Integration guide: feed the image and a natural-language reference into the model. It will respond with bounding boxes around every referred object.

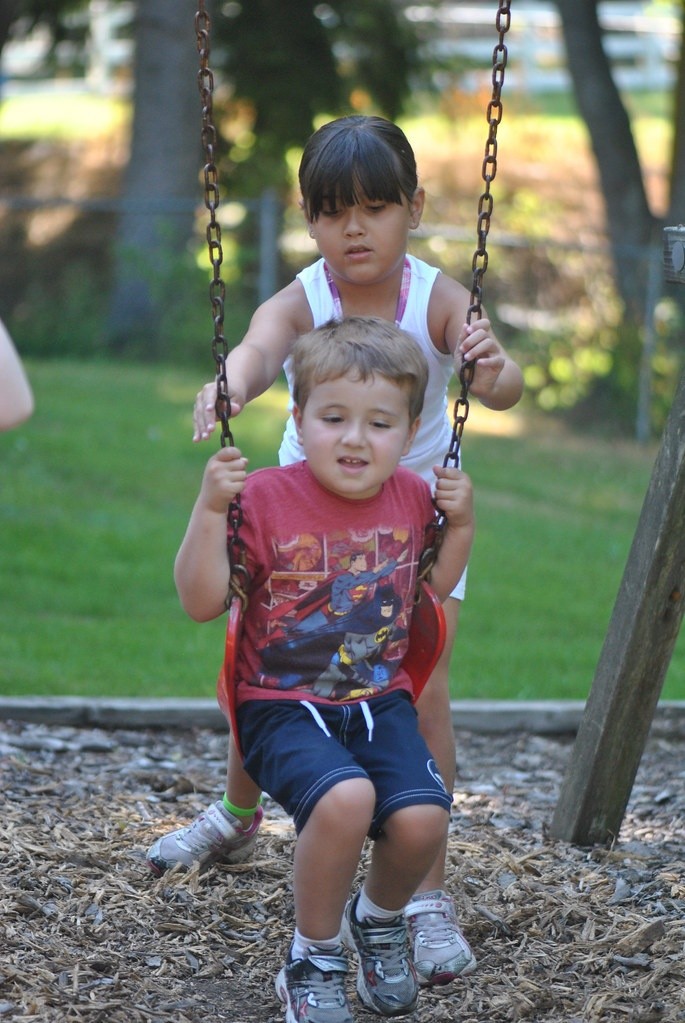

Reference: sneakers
[274,939,351,1023]
[340,891,419,1017]
[144,801,262,875]
[404,889,476,986]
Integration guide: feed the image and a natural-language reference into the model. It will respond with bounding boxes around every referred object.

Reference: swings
[191,0,513,760]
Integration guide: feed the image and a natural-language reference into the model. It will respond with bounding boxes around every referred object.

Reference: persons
[0,318,36,434]
[145,113,523,990]
[172,316,474,1023]
